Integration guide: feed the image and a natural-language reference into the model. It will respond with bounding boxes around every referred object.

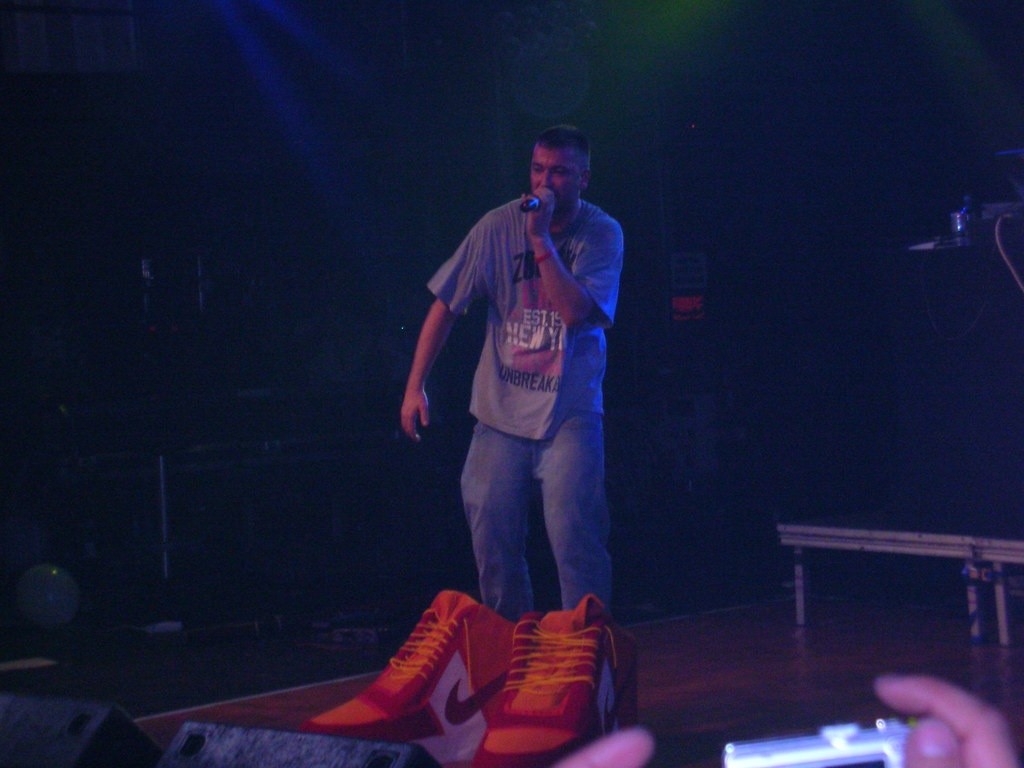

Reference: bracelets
[534,248,554,263]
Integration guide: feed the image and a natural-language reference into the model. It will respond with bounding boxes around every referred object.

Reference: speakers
[0,693,442,768]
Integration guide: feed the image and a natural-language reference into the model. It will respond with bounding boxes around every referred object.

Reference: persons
[552,675,1020,768]
[400,126,624,617]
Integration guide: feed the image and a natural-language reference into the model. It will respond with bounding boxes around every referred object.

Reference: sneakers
[297,589,516,742]
[472,593,638,768]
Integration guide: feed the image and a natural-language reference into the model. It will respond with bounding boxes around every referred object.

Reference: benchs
[775,514,1024,647]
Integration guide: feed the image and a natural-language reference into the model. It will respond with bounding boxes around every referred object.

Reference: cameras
[721,722,909,768]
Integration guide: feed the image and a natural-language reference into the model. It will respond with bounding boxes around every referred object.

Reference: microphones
[520,187,555,212]
